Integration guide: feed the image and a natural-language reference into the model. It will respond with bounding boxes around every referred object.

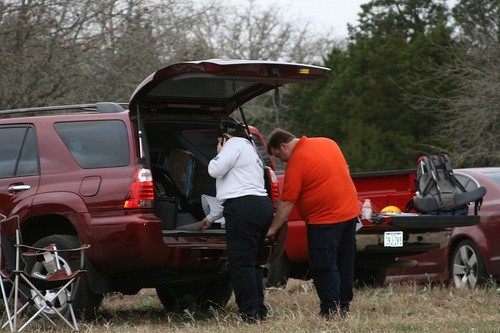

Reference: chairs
[0,215,91,333]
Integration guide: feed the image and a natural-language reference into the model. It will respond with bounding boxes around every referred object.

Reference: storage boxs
[156,196,178,230]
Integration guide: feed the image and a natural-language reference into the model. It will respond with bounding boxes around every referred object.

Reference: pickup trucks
[242,124,487,290]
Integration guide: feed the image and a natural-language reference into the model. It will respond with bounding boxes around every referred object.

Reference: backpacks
[405,152,487,215]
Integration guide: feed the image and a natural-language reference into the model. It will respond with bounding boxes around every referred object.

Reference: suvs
[0,58,331,323]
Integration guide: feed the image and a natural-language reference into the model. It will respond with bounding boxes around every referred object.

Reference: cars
[383,167,500,291]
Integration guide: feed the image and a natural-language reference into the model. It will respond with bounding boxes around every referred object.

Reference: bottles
[362,199,372,220]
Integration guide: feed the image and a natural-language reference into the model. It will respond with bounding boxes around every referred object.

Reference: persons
[200,117,275,325]
[264,129,363,321]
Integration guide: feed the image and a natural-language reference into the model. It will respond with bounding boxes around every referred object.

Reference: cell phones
[221,135,229,146]
